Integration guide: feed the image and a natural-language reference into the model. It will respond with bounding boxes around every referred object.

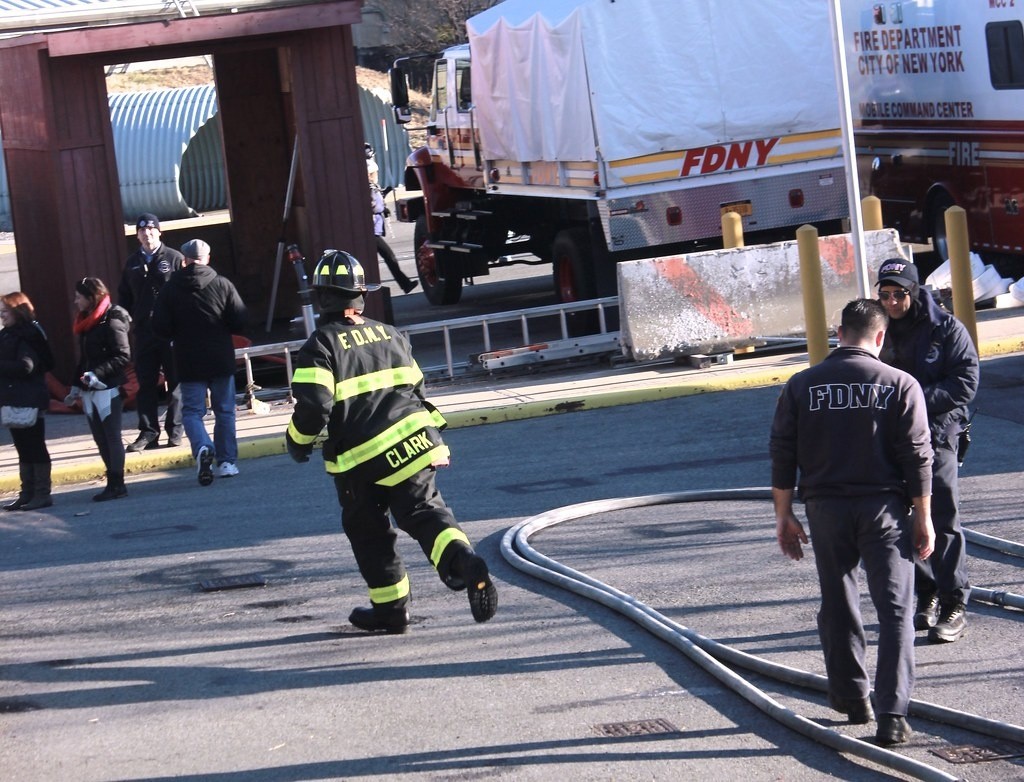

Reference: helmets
[310,249,381,291]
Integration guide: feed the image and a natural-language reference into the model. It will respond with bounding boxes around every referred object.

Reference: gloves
[287,440,312,463]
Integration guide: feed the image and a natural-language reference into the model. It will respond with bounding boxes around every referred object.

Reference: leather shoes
[876,714,912,742]
[463,555,499,623]
[349,607,410,634]
[848,697,876,723]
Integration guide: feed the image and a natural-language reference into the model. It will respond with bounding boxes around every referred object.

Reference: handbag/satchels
[1,406,38,426]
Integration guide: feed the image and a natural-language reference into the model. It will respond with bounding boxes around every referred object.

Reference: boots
[3,463,53,510]
[94,470,128,501]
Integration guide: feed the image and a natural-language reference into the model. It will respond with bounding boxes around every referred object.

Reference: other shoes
[404,281,418,293]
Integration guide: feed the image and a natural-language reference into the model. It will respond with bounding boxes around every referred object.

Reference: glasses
[878,288,909,300]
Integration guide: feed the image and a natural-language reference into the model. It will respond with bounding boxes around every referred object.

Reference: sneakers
[913,593,941,629]
[127,437,159,452]
[168,434,182,446]
[928,603,967,642]
[197,446,214,487]
[218,462,239,475]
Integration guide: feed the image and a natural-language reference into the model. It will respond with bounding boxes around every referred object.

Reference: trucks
[392,0,861,324]
[836,0,1024,251]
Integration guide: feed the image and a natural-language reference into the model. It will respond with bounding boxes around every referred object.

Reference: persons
[770,299,935,742]
[154,238,248,486]
[287,250,499,632]
[366,157,418,293]
[876,258,979,640]
[119,213,187,450]
[0,278,132,511]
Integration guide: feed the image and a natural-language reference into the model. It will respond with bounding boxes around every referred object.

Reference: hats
[181,239,210,259]
[136,213,160,232]
[366,159,379,173]
[874,258,919,290]
